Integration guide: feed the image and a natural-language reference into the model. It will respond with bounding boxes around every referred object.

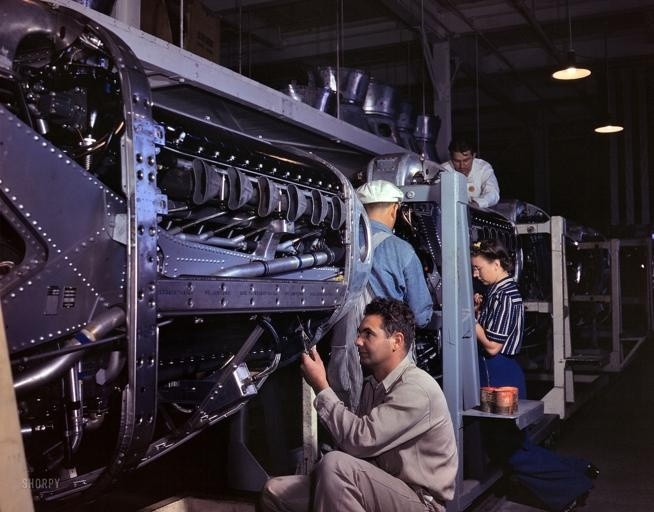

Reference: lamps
[548,0,594,82]
[589,31,624,135]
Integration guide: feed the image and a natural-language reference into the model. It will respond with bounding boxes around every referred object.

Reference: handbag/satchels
[506,445,595,511]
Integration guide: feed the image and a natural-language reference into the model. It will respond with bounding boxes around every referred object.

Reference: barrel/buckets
[480,387,497,412]
[498,387,519,412]
[496,390,513,416]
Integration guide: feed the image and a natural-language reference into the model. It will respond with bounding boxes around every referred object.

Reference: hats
[355,180,404,205]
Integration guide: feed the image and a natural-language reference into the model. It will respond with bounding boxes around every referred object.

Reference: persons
[261,296,459,511]
[470,237,527,400]
[432,138,502,241]
[326,179,434,415]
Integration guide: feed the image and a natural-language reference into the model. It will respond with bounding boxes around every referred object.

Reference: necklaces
[488,270,507,296]
